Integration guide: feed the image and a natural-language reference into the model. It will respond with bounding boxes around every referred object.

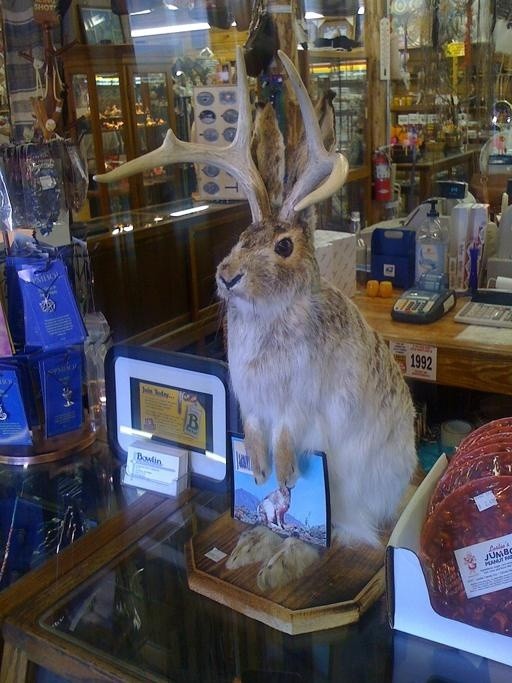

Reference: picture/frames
[76,2,127,45]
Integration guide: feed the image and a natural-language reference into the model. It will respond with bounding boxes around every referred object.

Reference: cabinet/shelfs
[60,43,187,220]
[388,39,511,214]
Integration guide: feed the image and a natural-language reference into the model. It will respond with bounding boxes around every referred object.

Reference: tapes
[441,419,472,448]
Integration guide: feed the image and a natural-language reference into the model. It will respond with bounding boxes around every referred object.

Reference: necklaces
[49,367,75,408]
[0,381,13,420]
[30,274,66,313]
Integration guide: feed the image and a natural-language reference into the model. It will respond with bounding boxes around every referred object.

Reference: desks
[349,283,511,396]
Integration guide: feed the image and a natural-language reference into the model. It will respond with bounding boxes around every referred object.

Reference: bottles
[348,211,368,291]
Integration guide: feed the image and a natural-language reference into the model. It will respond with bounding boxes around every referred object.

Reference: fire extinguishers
[372,144,395,201]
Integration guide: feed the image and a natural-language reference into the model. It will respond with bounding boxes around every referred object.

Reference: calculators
[455,288,512,326]
